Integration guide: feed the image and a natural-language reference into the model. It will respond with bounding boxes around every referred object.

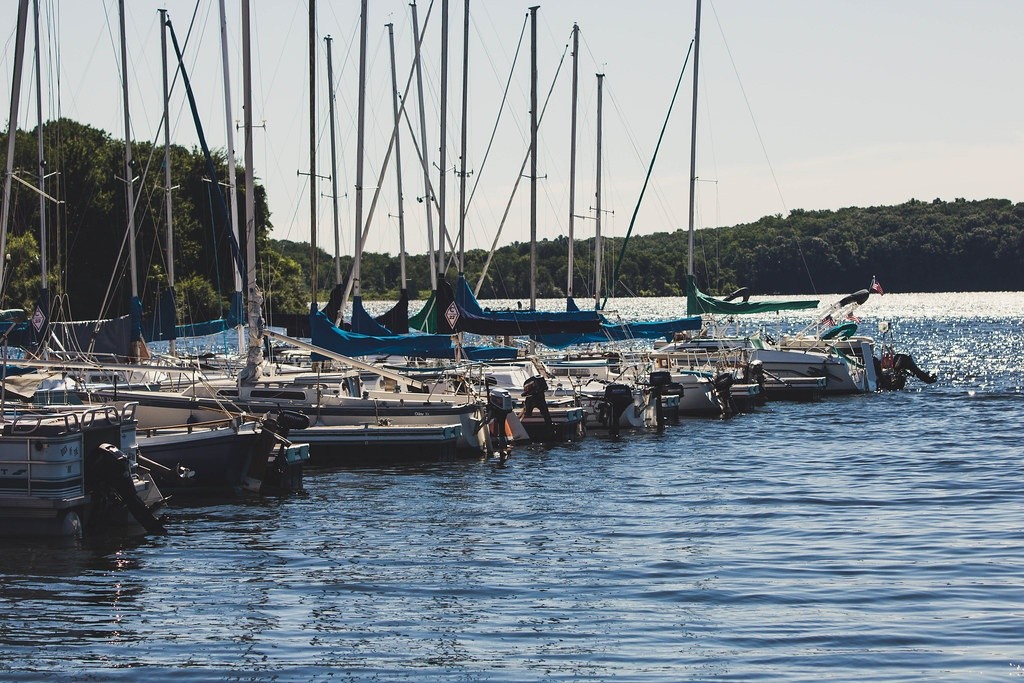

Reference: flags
[871,279,884,295]
[822,314,835,328]
[845,312,862,323]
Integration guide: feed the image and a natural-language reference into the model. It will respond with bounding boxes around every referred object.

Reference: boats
[0,0,931,534]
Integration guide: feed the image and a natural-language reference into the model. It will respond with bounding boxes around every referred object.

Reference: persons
[522,373,556,435]
